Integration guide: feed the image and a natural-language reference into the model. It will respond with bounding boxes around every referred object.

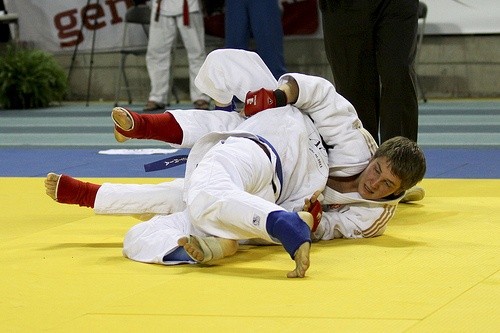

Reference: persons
[224,0,287,103]
[142,0,211,110]
[319,0,426,203]
[123,48,329,278]
[45,73,426,246]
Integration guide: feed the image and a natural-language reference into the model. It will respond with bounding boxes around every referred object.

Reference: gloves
[303,199,322,233]
[245,87,276,118]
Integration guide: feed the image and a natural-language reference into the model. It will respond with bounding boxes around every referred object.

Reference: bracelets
[274,89,287,107]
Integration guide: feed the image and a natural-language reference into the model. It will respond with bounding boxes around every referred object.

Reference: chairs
[410,1,428,105]
[114,5,180,107]
